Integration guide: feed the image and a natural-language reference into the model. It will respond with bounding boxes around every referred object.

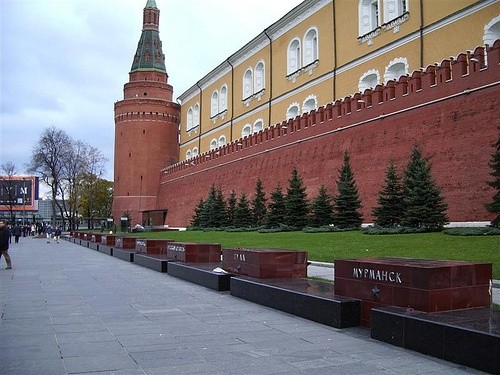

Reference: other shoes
[5,267,12,270]
[57,242,59,244]
[47,241,50,244]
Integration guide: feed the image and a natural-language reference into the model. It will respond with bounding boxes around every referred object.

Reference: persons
[5,221,61,243]
[0,220,12,269]
